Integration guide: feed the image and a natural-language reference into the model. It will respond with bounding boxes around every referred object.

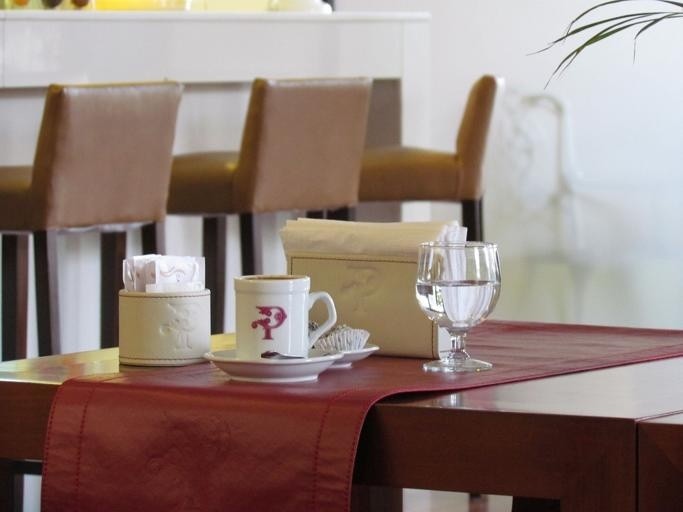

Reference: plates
[313,342,380,369]
[204,348,342,383]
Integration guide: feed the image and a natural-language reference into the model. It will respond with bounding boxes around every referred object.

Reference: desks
[0,12,439,350]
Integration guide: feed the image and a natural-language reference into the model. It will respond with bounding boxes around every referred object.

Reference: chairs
[0,81,183,359]
[142,74,373,335]
[325,74,498,242]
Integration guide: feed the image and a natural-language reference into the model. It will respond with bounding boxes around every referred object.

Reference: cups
[234,274,337,360]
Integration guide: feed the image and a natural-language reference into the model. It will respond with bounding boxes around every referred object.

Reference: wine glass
[414,241,502,374]
